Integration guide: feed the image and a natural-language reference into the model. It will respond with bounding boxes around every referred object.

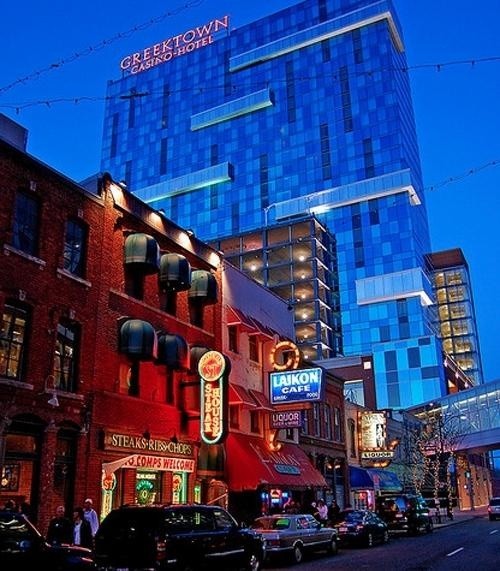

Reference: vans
[374,493,433,534]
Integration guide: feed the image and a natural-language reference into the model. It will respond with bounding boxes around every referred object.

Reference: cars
[326,510,391,547]
[239,514,338,561]
[0,511,94,570]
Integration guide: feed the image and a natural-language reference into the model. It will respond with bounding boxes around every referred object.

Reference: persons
[82,498,99,539]
[46,506,73,545]
[71,508,92,550]
[3,500,17,513]
[18,503,31,519]
[271,496,340,527]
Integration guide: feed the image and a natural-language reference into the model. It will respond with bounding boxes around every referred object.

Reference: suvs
[487,497,500,521]
[94,506,267,571]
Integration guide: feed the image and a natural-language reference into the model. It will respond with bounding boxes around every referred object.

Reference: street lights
[438,416,469,517]
[326,462,342,504]
